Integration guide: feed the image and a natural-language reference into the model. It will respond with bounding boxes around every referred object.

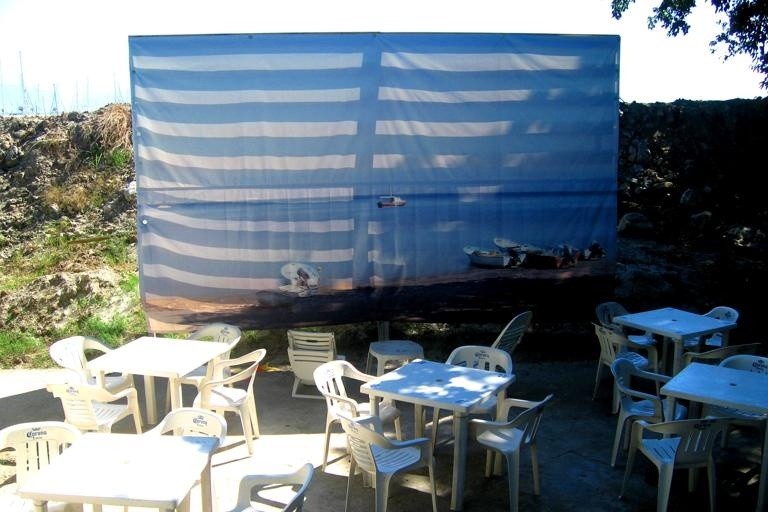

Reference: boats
[280,260,322,293]
[463,236,607,269]
[256,286,305,309]
[378,184,405,208]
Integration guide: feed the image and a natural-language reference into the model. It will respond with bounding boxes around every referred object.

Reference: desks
[359,358,516,511]
[85,336,229,434]
[19,433,219,512]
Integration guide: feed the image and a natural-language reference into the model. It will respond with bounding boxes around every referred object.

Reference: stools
[366,339,424,403]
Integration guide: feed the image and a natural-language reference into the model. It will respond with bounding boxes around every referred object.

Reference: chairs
[121,408,227,512]
[165,323,241,414]
[287,331,346,401]
[593,294,767,512]
[458,310,533,367]
[0,421,83,512]
[50,336,144,428]
[313,361,402,489]
[193,348,267,455]
[229,463,314,512]
[467,394,554,511]
[337,414,437,512]
[432,345,512,438]
[36,368,141,434]
